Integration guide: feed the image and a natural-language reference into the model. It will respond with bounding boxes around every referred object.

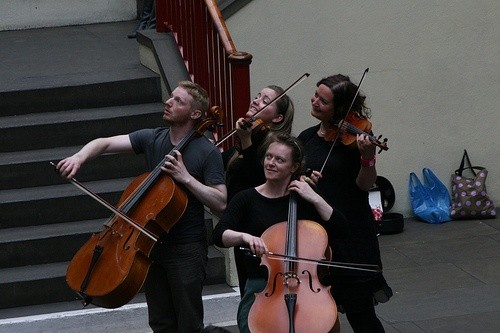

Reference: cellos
[246,170,341,333]
[65,105,224,310]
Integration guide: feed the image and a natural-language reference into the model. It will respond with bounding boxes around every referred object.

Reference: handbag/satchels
[409,167,451,223]
[450,149,496,219]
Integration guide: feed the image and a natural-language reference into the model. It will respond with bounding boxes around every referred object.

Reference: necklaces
[320,123,326,135]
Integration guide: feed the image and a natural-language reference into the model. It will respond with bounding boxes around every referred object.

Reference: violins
[237,116,269,144]
[323,108,390,154]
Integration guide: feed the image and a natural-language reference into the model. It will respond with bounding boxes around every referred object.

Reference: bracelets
[361,154,376,167]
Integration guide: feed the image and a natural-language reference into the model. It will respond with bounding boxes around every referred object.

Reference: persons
[296,75,393,333]
[56,81,228,333]
[208,85,294,300]
[210,130,342,333]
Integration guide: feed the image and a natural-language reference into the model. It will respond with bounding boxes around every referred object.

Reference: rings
[305,177,310,181]
